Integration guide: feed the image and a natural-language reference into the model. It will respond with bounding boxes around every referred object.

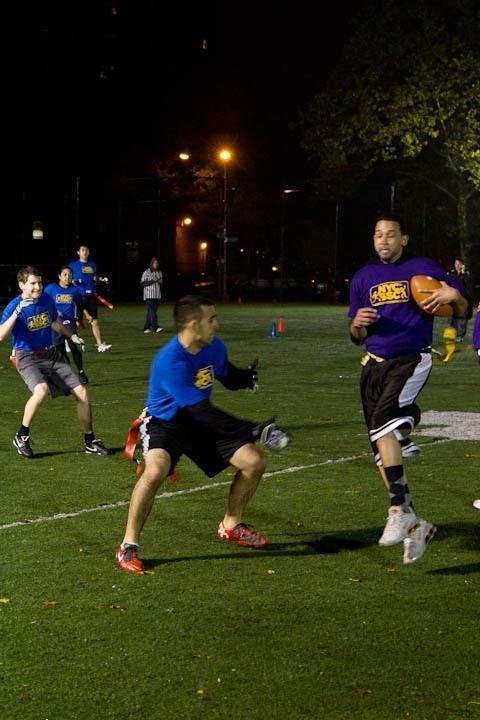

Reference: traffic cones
[278,317,284,333]
[270,321,279,339]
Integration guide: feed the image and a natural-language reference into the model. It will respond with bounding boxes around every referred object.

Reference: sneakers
[115,542,146,573]
[378,505,418,547]
[84,439,112,456]
[402,519,438,564]
[98,343,112,353]
[143,329,152,334]
[79,371,88,385]
[12,430,36,458]
[156,328,163,332]
[216,522,269,548]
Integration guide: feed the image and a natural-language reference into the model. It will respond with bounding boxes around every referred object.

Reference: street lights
[217,144,237,303]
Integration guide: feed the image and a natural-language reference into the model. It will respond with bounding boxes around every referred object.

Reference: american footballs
[411,275,453,317]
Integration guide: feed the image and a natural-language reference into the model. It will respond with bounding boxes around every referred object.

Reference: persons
[0,264,113,458]
[344,213,473,568]
[115,293,269,575]
[473,302,480,510]
[139,256,165,334]
[42,264,94,385]
[67,244,113,354]
[444,256,473,342]
